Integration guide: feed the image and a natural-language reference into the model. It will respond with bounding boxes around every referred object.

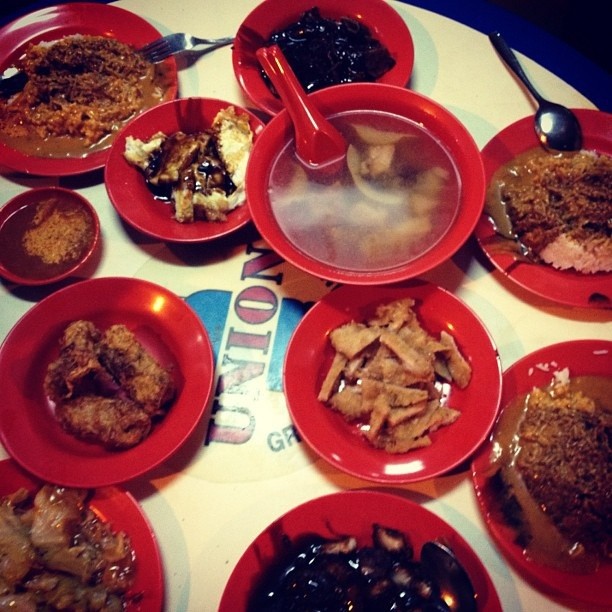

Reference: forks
[139,30,235,62]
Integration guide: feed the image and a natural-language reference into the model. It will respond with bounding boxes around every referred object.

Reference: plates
[103,95,269,246]
[0,1,179,180]
[230,0,417,119]
[281,275,505,486]
[244,80,490,287]
[474,106,612,312]
[0,456,167,611]
[0,275,216,491]
[0,183,103,295]
[469,337,612,611]
[215,486,506,611]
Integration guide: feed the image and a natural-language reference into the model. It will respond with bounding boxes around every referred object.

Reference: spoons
[485,25,587,158]
[255,45,350,186]
